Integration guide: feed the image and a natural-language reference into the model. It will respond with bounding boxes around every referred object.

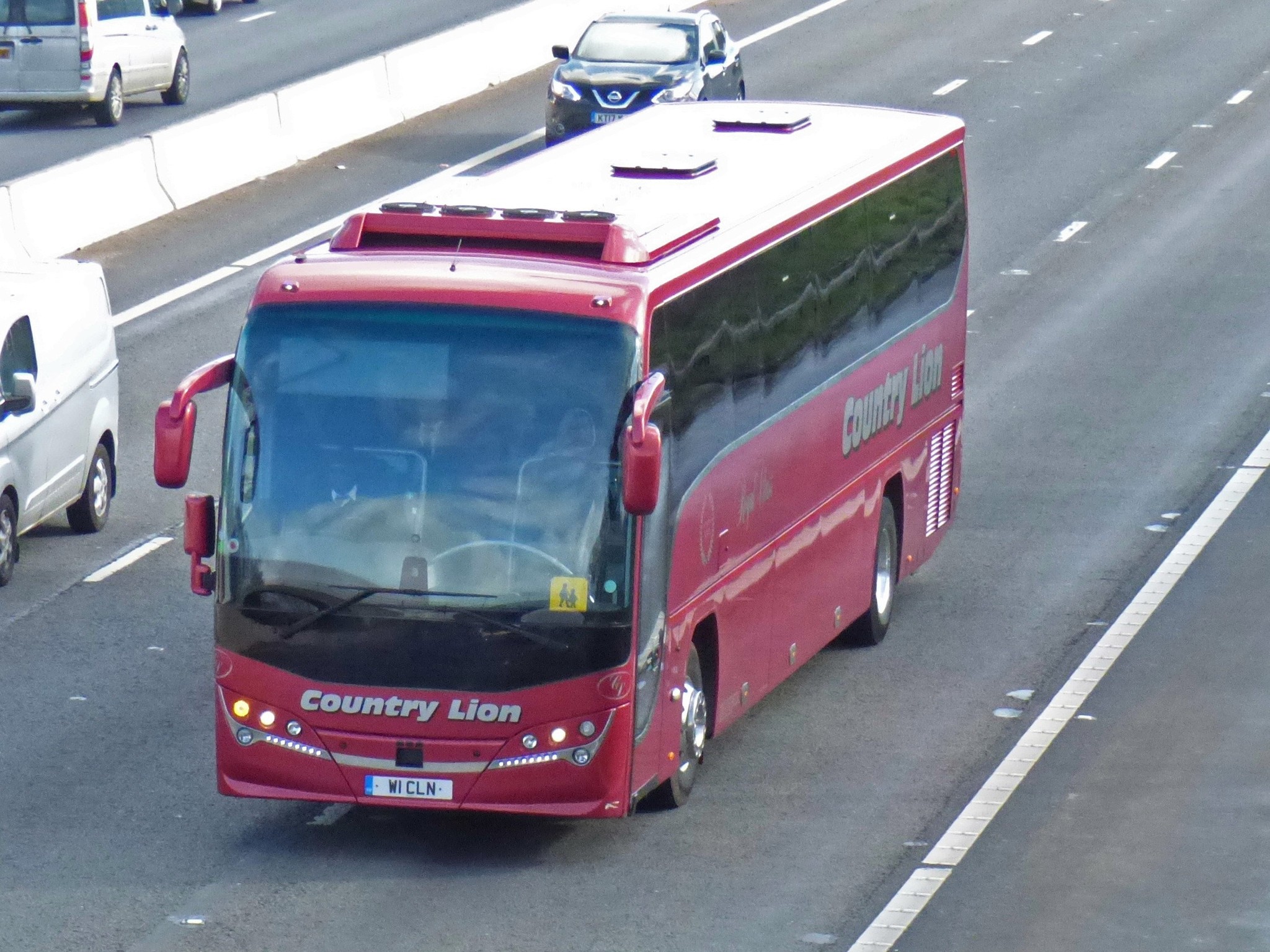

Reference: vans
[0,0,191,127]
[0,256,122,587]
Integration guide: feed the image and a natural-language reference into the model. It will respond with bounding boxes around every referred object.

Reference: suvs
[545,8,746,148]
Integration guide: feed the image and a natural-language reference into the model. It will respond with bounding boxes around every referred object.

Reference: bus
[155,101,969,818]
